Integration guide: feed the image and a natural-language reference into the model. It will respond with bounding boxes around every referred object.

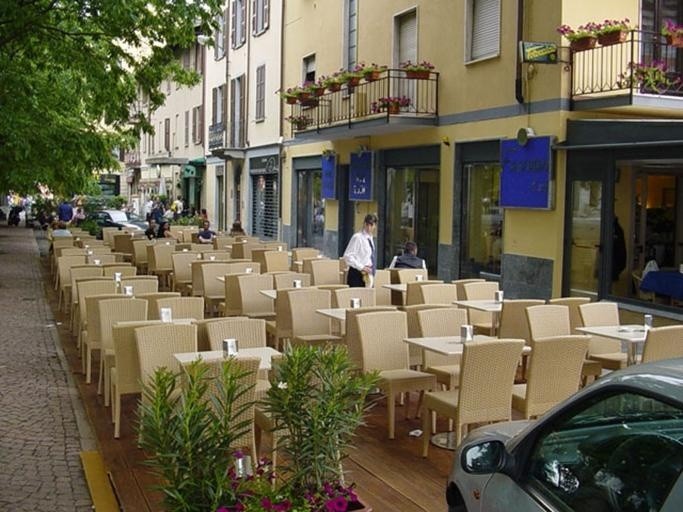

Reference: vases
[664,34,683,49]
[595,30,626,45]
[570,35,595,51]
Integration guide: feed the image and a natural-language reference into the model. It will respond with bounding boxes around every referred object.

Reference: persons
[342,214,378,286]
[404,197,413,227]
[6,182,244,244]
[313,197,324,236]
[388,242,426,268]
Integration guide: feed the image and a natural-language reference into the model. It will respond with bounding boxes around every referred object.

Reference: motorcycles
[5,205,23,227]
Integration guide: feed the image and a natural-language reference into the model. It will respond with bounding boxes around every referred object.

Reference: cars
[478,207,503,234]
[440,353,681,510]
[88,209,147,237]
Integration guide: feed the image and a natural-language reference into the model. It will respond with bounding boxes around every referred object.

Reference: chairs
[335,266,681,459]
[47,223,346,489]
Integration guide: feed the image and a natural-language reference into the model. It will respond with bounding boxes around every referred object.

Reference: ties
[367,238,378,277]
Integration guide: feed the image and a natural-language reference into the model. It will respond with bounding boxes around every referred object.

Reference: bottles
[643,314,653,331]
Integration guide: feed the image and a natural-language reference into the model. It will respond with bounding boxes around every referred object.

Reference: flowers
[276,57,433,129]
[556,21,599,38]
[615,61,680,98]
[592,18,633,33]
[660,21,683,35]
[139,339,373,512]
